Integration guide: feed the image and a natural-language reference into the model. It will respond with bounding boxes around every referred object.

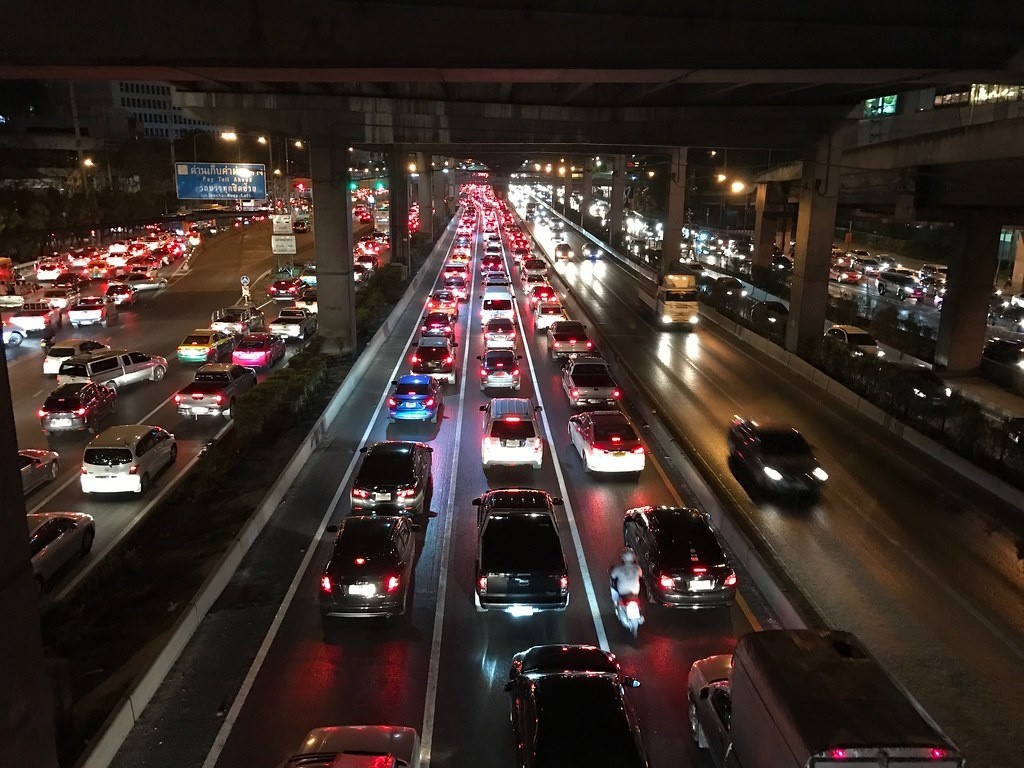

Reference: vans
[686,628,967,768]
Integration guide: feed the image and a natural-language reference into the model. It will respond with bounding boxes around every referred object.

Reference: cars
[82,424,178,496]
[348,438,433,517]
[730,415,829,499]
[286,723,421,768]
[27,511,95,588]
[478,396,545,471]
[16,448,60,499]
[318,513,421,620]
[503,643,648,768]
[0,184,1024,481]
[472,488,570,614]
[622,504,736,609]
[567,411,645,473]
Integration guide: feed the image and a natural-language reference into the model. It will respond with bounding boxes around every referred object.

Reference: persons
[41,319,56,346]
[611,548,642,615]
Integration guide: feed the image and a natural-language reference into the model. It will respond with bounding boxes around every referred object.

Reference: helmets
[624,551,633,560]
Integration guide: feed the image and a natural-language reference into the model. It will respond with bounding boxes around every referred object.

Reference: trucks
[638,262,700,330]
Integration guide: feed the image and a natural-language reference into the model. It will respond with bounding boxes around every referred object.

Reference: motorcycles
[609,565,645,637]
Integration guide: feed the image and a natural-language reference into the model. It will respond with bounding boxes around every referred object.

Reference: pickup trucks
[560,356,622,408]
[270,307,317,342]
[174,361,256,421]
[547,321,592,361]
